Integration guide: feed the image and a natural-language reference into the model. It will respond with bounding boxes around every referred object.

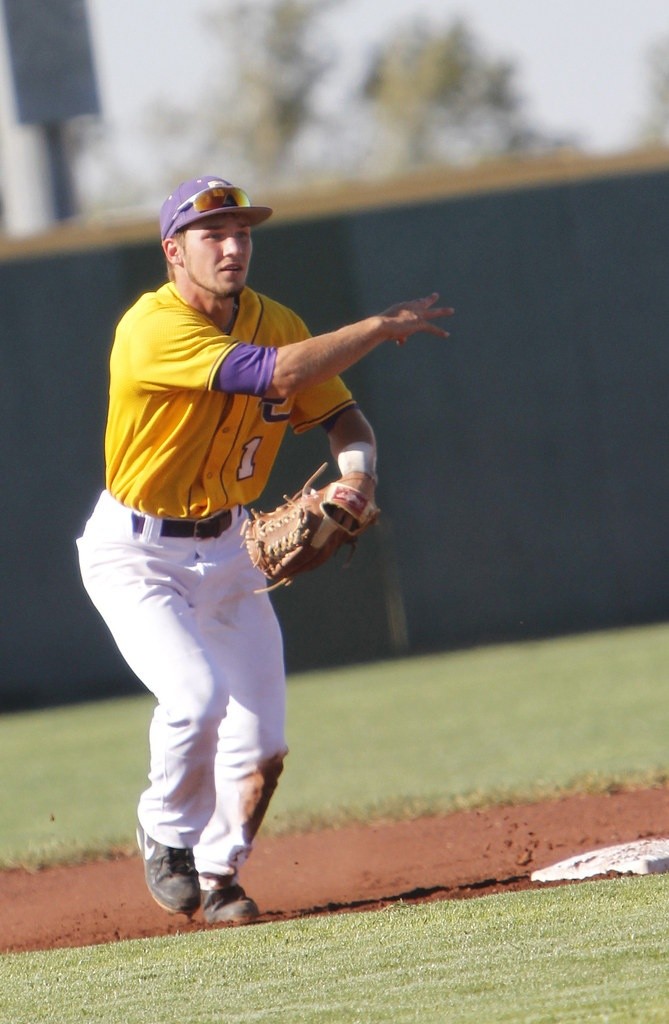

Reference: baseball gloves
[243,468,384,584]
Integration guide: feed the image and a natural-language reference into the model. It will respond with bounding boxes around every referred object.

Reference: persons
[73,177,456,920]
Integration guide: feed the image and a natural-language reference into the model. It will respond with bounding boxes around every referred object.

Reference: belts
[132,505,242,542]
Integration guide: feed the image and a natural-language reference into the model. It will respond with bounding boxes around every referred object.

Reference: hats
[158,175,274,238]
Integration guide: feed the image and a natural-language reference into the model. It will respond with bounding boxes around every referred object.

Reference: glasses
[164,184,252,241]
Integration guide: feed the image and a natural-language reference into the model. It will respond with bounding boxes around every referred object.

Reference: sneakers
[135,820,200,913]
[199,886,260,923]
